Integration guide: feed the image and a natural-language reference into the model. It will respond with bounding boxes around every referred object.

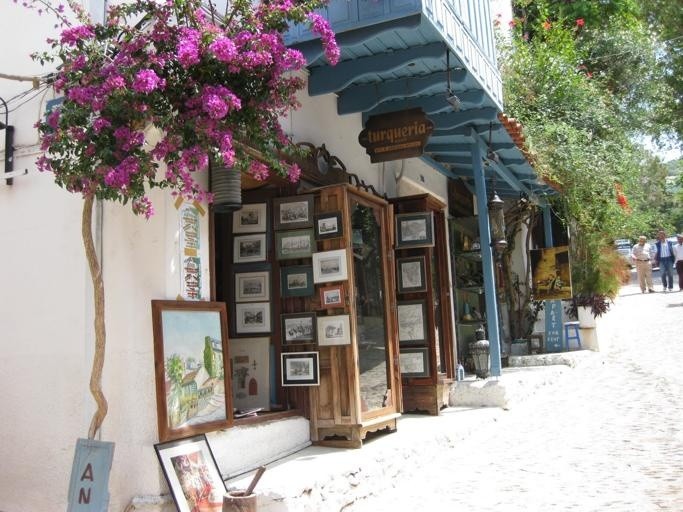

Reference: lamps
[488,171,508,214]
[485,101,498,166]
[444,38,460,108]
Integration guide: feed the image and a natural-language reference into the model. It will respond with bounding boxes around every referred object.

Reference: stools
[526,333,542,356]
[563,318,580,352]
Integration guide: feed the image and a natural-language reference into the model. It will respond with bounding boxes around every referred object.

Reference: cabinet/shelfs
[449,213,493,368]
[290,180,408,452]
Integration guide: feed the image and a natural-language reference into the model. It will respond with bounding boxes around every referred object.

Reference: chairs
[383,186,458,417]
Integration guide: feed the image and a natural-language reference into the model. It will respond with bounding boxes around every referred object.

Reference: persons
[631,236,656,294]
[671,234,683,292]
[654,230,675,292]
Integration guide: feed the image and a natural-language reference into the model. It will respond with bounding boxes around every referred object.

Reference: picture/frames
[390,208,438,379]
[221,199,354,415]
[150,434,227,511]
[148,295,234,441]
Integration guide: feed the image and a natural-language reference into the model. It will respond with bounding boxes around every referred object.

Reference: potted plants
[566,292,609,329]
